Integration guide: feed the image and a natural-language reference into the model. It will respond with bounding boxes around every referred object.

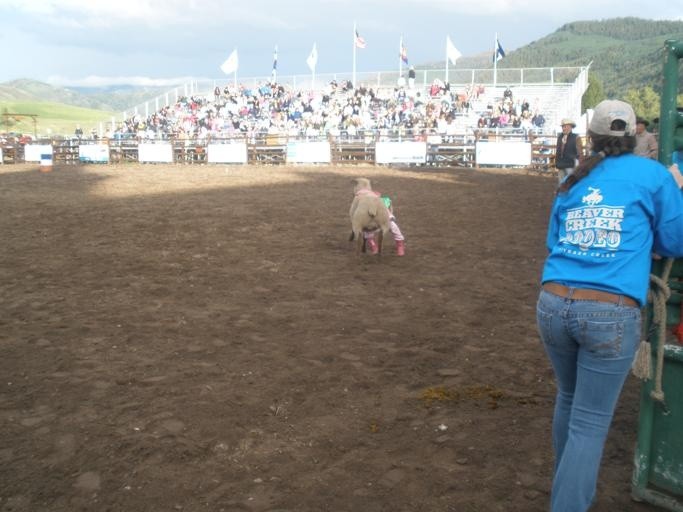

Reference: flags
[401,42,408,64]
[354,26,367,48]
[447,37,461,65]
[306,43,318,73]
[219,47,239,75]
[270,42,278,79]
[491,36,507,64]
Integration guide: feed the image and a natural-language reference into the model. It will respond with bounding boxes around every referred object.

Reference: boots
[397,240,404,256]
[367,237,379,254]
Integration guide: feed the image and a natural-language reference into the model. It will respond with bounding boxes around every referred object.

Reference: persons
[632,116,658,160]
[73,78,552,170]
[362,189,406,257]
[533,97,683,512]
[555,118,583,185]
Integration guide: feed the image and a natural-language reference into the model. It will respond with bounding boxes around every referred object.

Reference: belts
[542,280,638,308]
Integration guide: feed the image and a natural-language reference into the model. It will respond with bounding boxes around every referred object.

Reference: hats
[636,117,650,125]
[561,119,576,128]
[588,98,636,137]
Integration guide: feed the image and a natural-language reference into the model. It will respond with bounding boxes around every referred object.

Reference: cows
[349,176,391,260]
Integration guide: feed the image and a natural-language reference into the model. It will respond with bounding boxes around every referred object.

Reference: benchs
[448,84,567,130]
